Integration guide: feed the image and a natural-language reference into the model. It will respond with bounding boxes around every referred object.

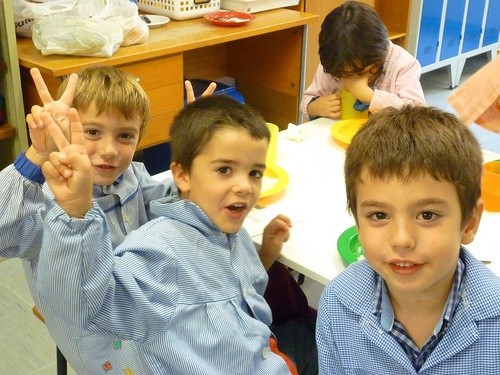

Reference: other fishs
[214,15,257,23]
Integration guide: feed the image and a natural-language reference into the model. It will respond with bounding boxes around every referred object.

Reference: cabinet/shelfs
[0,0,500,172]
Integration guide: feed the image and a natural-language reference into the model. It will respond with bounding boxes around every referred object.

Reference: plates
[203,11,255,27]
[139,14,171,28]
[258,163,288,198]
[337,226,363,265]
[331,118,369,145]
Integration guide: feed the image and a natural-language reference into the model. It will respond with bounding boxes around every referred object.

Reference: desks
[151,111,500,289]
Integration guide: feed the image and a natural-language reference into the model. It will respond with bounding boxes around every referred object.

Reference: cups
[265,122,279,164]
[479,162,500,212]
[341,88,368,119]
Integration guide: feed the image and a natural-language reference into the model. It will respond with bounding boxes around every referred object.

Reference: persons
[314,103,500,375]
[299,0,427,119]
[34,94,317,375]
[0,66,317,327]
